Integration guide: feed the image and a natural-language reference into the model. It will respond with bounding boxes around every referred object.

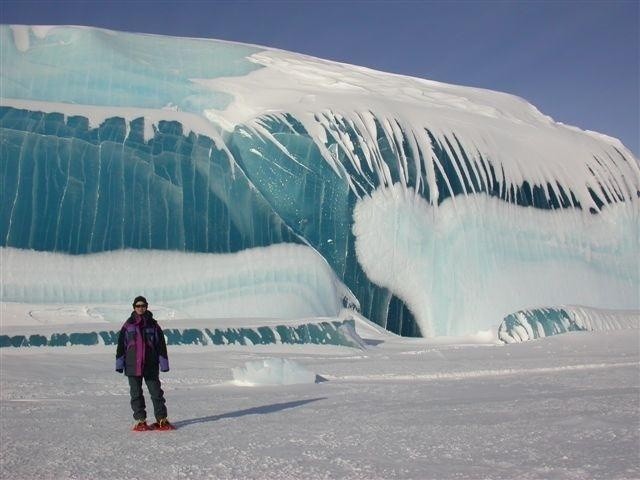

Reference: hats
[133,296,148,309]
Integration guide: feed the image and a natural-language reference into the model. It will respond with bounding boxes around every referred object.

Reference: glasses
[135,304,147,308]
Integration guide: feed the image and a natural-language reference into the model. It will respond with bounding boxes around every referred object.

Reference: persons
[115,296,176,432]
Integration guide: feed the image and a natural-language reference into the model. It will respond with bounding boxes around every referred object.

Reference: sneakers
[136,419,147,431]
[156,416,170,430]
